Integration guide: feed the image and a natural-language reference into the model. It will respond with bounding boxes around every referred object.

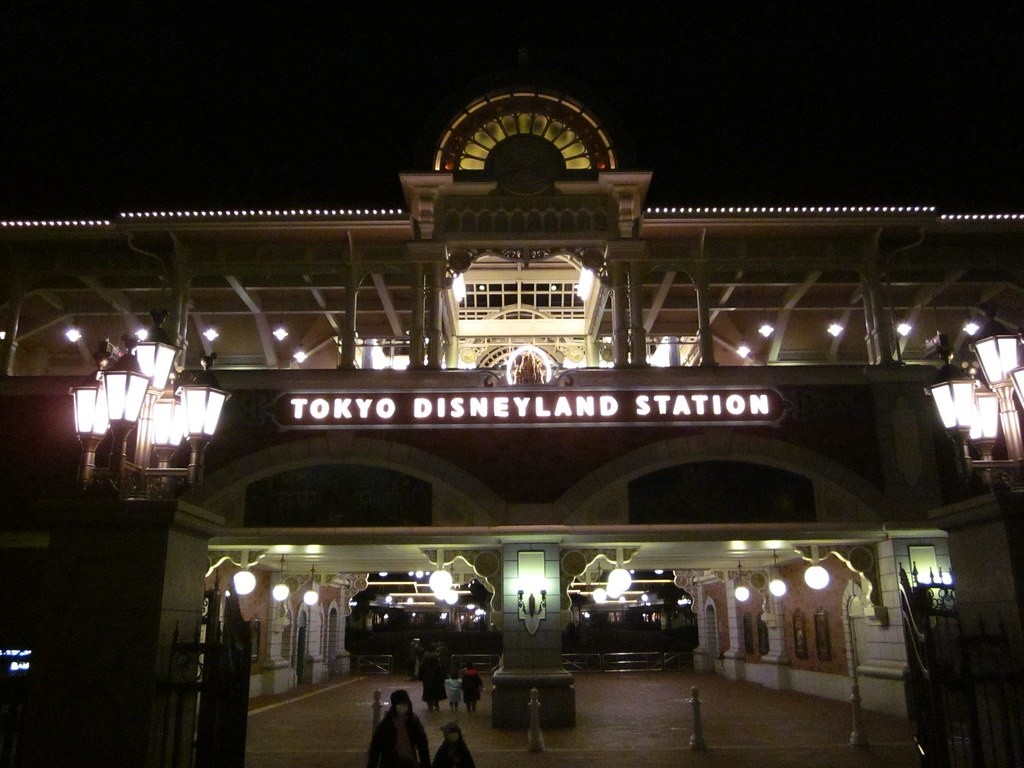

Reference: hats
[390,690,409,704]
[439,721,460,734]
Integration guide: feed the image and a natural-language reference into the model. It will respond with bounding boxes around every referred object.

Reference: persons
[407,640,481,712]
[432,720,475,768]
[367,690,432,768]
